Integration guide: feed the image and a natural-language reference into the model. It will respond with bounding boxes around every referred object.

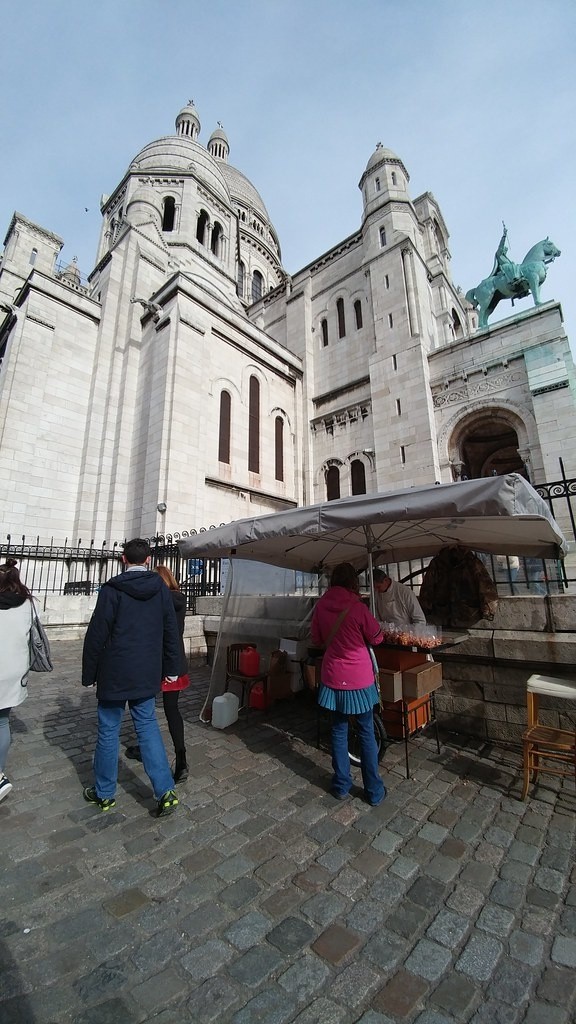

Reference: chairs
[519,723,576,800]
[224,641,270,712]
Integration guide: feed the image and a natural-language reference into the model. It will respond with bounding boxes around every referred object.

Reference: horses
[464,236,561,328]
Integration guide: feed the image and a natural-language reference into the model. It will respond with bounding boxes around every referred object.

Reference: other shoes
[127,746,144,761]
[370,787,388,805]
[173,751,189,784]
[336,793,348,800]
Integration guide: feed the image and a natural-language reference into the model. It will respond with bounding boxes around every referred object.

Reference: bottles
[249,681,271,710]
[211,693,240,730]
[238,646,260,677]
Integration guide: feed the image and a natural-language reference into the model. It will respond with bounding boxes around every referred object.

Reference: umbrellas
[175,470,570,621]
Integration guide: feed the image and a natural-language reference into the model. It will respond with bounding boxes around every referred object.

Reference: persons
[310,560,388,806]
[368,567,429,628]
[81,538,179,817]
[0,558,41,802]
[126,565,191,784]
[494,227,526,289]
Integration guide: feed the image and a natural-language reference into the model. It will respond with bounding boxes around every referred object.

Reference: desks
[525,673,576,773]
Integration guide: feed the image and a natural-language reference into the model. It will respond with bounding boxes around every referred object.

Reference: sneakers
[84,786,116,811]
[0,772,14,801]
[159,791,180,818]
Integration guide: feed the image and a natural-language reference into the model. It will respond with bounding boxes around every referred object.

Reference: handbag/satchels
[29,598,53,672]
